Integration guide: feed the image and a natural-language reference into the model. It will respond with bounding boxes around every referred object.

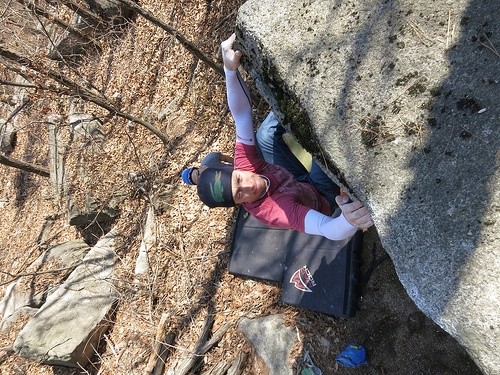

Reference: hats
[182,166,197,185]
[197,167,235,209]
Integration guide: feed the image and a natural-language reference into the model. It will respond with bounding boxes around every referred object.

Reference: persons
[182,152,234,187]
[196,32,374,241]
